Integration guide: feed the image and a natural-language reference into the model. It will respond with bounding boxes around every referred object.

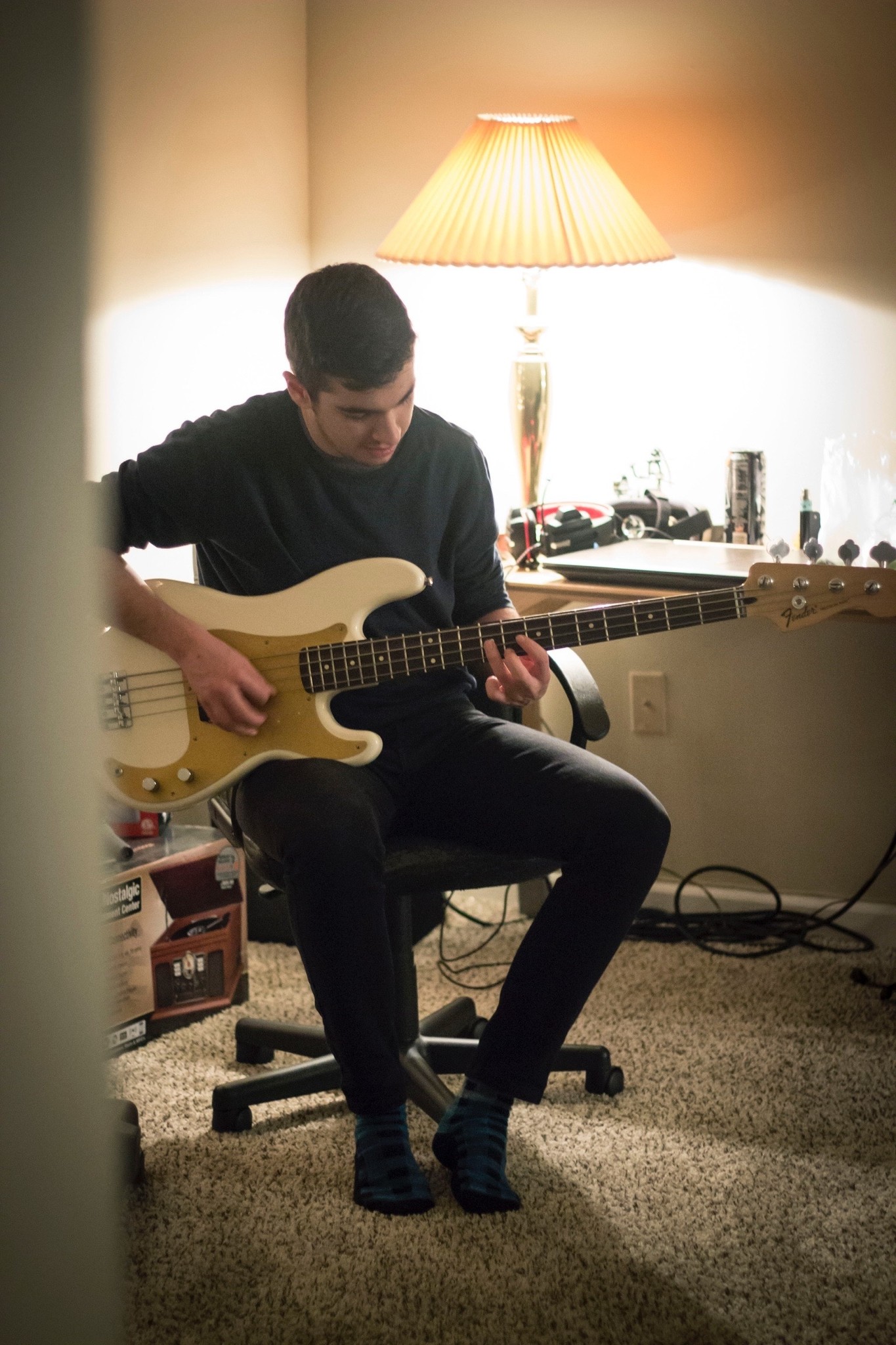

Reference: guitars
[96,537,896,814]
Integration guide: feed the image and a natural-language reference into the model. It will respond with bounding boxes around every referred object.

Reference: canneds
[725,448,768,547]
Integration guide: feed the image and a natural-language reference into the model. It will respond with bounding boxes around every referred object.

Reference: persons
[84,262,670,1215]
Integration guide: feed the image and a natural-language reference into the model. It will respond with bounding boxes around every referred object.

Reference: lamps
[375,117,676,508]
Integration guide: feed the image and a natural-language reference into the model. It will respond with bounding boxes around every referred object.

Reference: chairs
[203,642,624,1134]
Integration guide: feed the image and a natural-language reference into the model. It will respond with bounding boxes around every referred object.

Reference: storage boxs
[100,823,251,1056]
